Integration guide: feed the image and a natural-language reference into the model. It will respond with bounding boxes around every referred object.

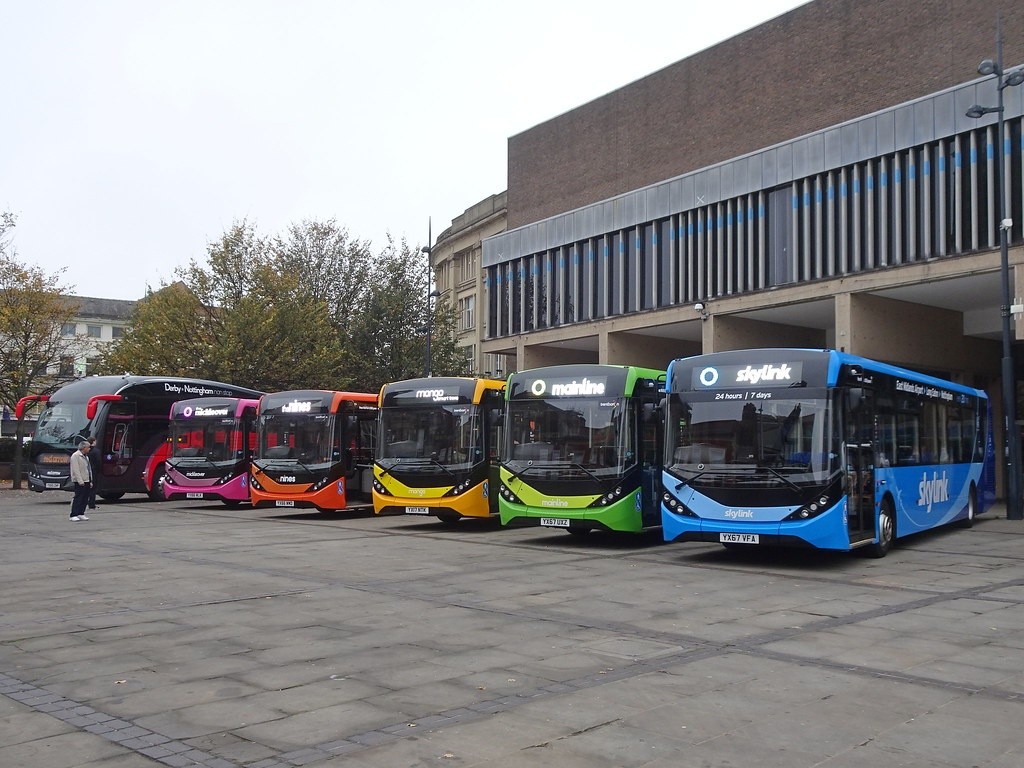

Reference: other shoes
[70,514,89,522]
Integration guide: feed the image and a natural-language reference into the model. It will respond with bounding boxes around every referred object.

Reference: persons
[68,441,93,522]
[87,435,100,510]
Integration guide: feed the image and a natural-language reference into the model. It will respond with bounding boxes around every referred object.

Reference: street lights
[964,62,1024,520]
[424,290,440,379]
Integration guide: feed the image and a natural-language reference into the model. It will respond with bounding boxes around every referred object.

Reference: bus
[164,399,252,507]
[250,389,375,513]
[650,346,995,561]
[14,372,265,503]
[372,376,510,524]
[495,365,665,538]
